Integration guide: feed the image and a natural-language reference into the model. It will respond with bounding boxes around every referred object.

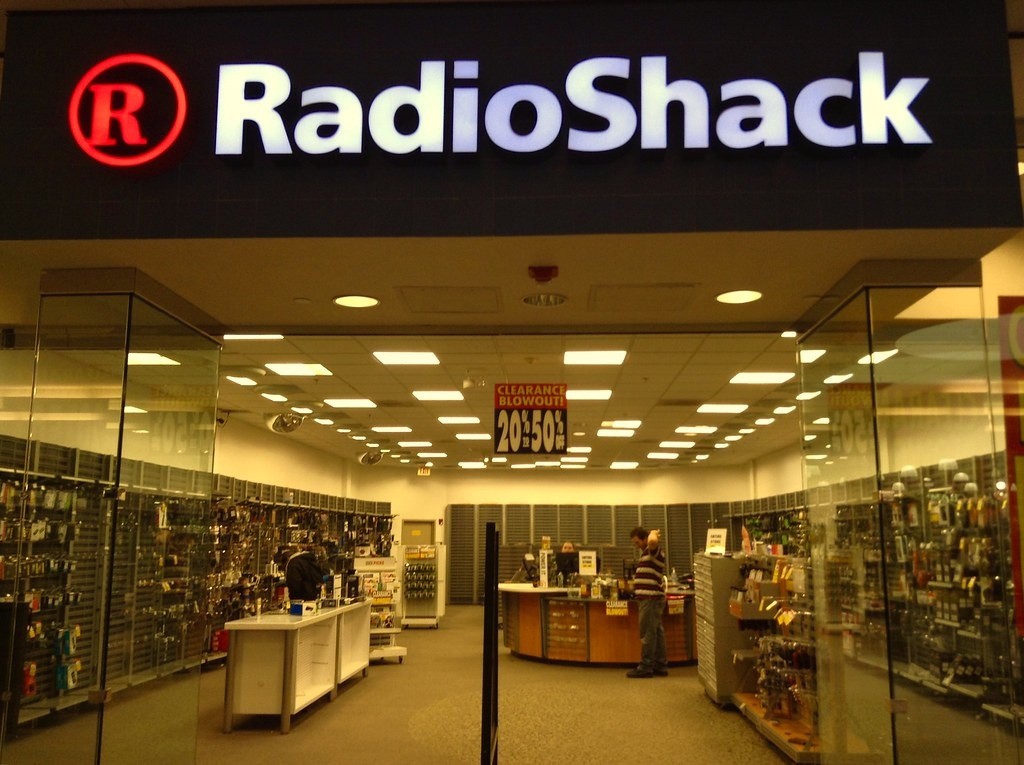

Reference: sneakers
[626,667,653,678]
[653,669,668,677]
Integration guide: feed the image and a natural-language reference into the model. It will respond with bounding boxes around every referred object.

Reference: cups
[541,535,550,549]
[292,601,317,615]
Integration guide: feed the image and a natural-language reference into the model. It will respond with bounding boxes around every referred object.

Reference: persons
[274,545,326,600]
[626,528,668,677]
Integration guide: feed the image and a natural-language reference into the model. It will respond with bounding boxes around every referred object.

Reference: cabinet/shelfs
[0,434,446,741]
[694,486,1024,765]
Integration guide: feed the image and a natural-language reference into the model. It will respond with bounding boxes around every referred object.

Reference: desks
[221,609,342,732]
[278,597,374,700]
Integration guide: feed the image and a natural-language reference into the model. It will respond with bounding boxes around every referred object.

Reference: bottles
[557,572,564,587]
